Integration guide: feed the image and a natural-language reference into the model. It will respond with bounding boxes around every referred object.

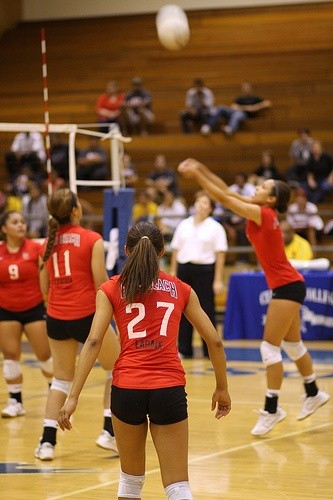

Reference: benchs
[0,0,333,269]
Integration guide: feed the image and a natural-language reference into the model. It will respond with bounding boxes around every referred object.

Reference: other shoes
[34,437,56,460]
[95,428,119,453]
[1,398,26,417]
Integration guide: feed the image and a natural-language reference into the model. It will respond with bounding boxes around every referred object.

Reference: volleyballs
[155,6,191,49]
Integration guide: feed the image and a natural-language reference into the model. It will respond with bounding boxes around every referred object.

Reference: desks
[219,270,332,360]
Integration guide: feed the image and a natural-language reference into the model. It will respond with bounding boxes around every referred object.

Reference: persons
[0,127,332,266]
[120,76,155,136]
[0,208,53,419]
[198,79,271,139]
[95,79,126,135]
[165,189,229,361]
[54,220,231,500]
[179,77,217,135]
[176,157,331,438]
[32,189,121,462]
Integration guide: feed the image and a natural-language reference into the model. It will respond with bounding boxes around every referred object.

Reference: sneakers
[296,389,330,420]
[252,407,286,436]
